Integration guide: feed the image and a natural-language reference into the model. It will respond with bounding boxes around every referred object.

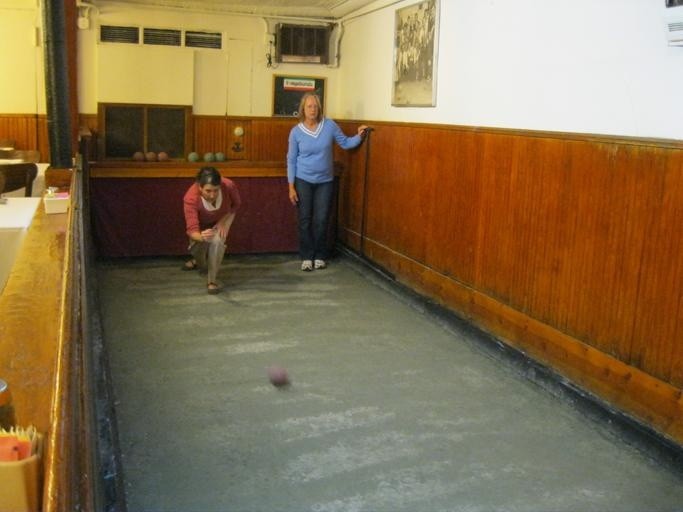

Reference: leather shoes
[181,259,198,270]
[207,282,219,294]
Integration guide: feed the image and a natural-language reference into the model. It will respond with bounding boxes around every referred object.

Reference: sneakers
[313,260,327,269]
[301,260,313,272]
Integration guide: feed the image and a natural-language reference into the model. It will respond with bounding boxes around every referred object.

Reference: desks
[0,163,51,197]
[0,197,68,292]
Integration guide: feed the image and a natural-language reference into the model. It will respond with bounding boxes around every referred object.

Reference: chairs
[0,163,38,197]
[0,149,40,163]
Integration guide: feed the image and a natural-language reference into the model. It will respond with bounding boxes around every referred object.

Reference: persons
[393,1,436,96]
[181,166,240,294]
[286,91,368,272]
